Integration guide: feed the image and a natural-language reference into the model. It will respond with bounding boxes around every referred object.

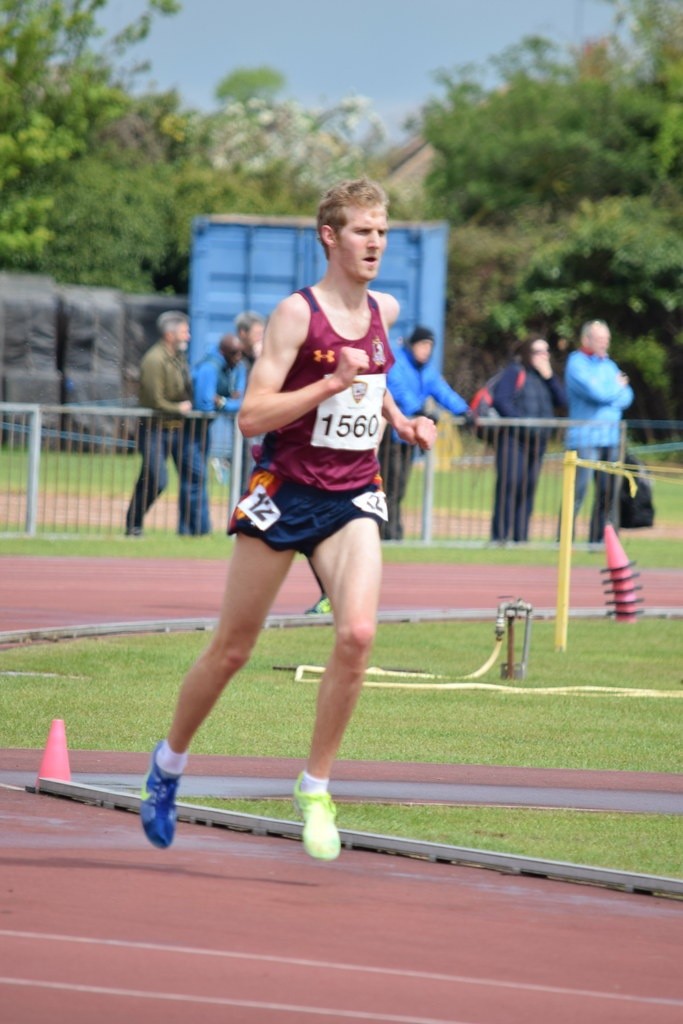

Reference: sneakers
[293,773,341,861]
[141,745,180,848]
[304,594,334,616]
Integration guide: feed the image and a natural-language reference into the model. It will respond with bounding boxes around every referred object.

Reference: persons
[140,181,436,859]
[479,336,564,541]
[376,327,475,540]
[558,319,655,542]
[125,311,267,537]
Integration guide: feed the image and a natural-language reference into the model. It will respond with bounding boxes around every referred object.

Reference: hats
[408,328,434,342]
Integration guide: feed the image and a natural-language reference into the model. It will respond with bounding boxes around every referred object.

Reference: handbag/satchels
[593,449,654,527]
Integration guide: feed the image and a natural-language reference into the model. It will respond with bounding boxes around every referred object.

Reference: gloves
[421,401,439,425]
[462,411,476,429]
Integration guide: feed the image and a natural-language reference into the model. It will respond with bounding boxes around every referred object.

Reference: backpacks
[469,370,527,442]
